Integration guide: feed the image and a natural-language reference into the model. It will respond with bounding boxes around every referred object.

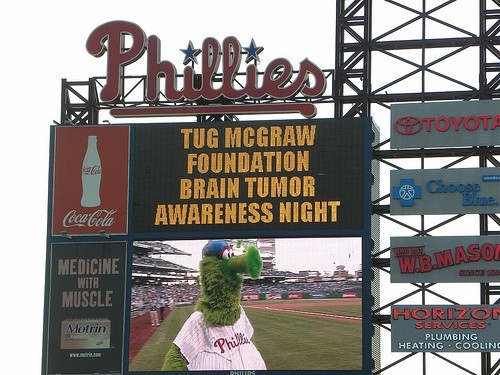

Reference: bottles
[80,135,102,208]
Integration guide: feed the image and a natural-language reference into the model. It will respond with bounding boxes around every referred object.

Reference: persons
[131,283,199,319]
[155,294,165,322]
[149,297,160,327]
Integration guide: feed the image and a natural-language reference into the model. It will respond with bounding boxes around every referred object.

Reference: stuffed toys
[159,239,268,371]
